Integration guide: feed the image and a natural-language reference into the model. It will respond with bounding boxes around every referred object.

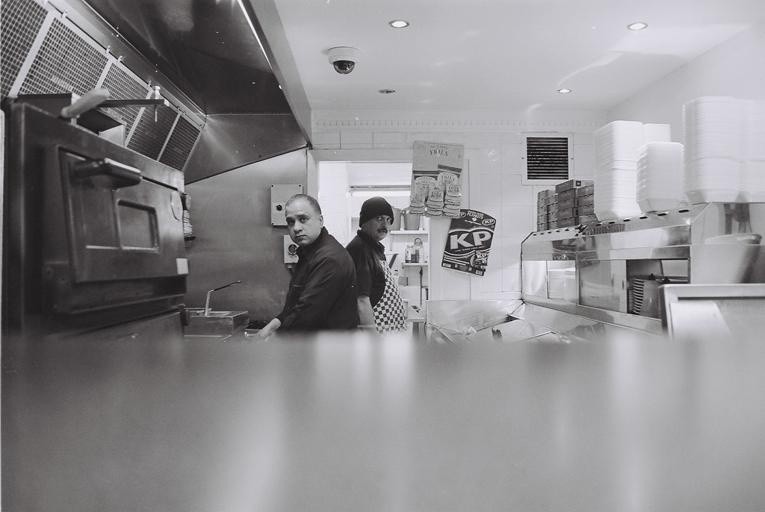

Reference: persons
[244,192,362,337]
[345,196,409,334]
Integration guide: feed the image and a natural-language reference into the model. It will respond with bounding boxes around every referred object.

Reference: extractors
[1,0,313,185]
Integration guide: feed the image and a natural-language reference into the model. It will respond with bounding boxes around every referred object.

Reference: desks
[404,306,426,340]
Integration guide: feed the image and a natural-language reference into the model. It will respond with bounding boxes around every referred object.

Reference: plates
[633,275,646,315]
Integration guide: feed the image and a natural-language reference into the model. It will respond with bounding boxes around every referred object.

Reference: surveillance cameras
[326,45,364,74]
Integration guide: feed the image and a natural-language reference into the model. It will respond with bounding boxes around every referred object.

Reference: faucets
[204,279,241,317]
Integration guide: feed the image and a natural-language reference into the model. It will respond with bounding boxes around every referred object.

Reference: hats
[359,198,393,227]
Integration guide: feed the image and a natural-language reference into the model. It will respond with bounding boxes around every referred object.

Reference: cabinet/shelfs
[383,230,429,266]
[517,200,725,335]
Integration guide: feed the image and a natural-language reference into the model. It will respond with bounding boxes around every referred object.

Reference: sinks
[190,310,248,318]
